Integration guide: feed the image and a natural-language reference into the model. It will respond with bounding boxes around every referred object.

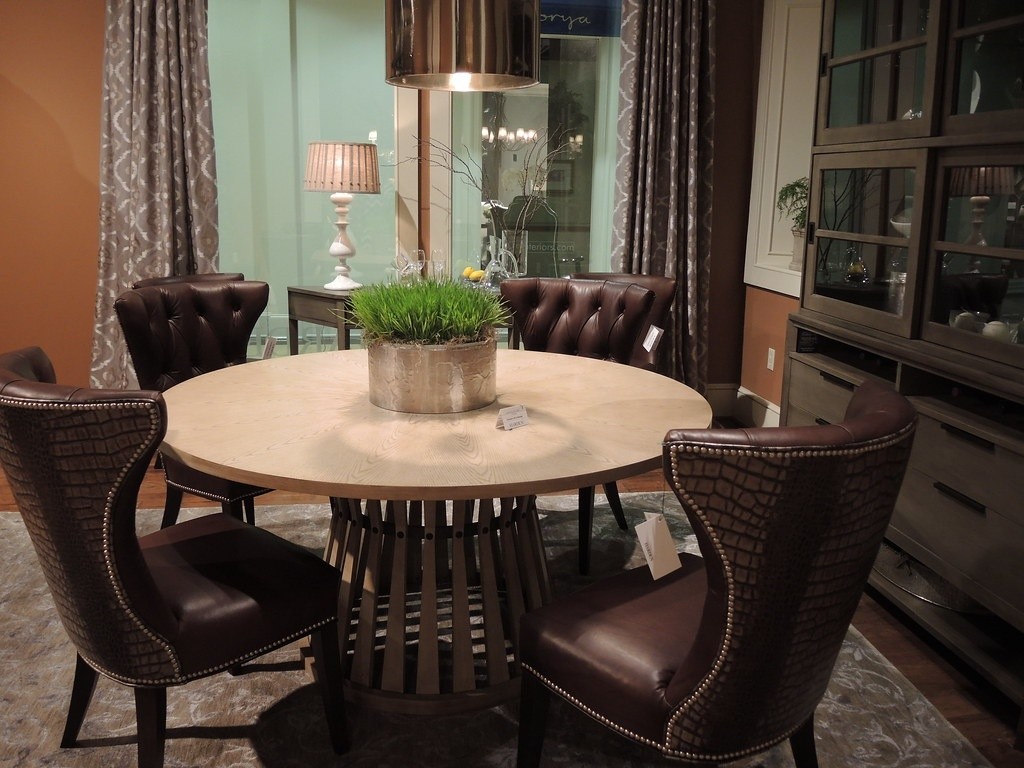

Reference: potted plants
[324,268,521,413]
[383,114,595,279]
[775,171,811,272]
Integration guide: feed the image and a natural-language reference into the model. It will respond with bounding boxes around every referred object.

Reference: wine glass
[384,248,446,285]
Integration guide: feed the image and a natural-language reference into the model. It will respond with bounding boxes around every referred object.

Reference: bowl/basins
[889,218,911,239]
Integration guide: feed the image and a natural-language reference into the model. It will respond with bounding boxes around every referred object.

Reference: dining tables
[568,268,680,370]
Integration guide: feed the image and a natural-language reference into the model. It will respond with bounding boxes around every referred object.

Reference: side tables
[282,283,369,357]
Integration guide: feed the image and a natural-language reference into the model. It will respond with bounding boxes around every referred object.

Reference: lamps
[479,94,537,156]
[384,0,542,93]
[302,139,382,294]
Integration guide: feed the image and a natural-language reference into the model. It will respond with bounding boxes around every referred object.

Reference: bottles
[477,235,518,293]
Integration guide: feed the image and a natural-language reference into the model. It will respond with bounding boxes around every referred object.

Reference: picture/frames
[547,159,575,193]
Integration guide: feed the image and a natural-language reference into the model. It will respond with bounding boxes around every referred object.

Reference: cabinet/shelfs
[770,0,1024,734]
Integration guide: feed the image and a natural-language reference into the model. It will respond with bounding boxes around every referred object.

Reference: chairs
[478,267,648,579]
[506,373,922,768]
[0,344,362,768]
[499,192,564,277]
[110,268,282,676]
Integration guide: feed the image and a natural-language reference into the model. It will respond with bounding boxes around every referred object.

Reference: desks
[155,340,718,722]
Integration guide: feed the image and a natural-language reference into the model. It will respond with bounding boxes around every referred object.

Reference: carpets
[0,491,999,768]
